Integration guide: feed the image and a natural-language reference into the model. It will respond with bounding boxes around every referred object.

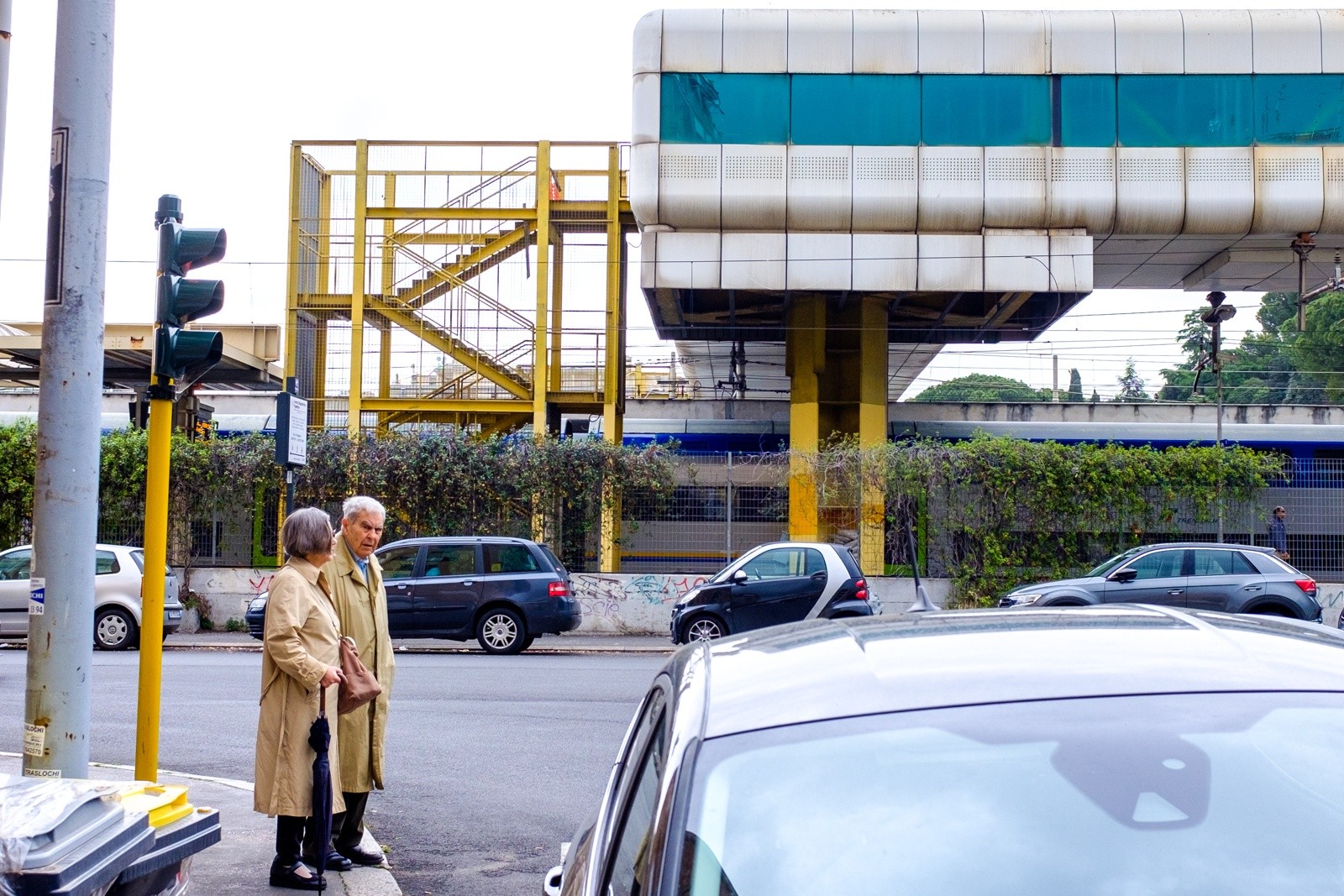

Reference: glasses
[1279,510,1285,513]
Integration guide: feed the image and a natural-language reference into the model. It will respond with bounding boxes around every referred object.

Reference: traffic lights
[151,221,225,385]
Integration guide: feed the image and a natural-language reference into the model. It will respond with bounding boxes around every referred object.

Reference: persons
[253,507,347,891]
[319,497,396,865]
[1268,506,1289,564]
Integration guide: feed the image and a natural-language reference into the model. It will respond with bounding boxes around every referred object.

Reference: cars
[542,599,1343,896]
[0,543,184,651]
[669,541,873,646]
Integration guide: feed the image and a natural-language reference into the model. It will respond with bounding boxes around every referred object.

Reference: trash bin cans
[0,774,222,896]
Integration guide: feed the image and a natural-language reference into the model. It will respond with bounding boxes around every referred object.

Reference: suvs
[244,535,583,655]
[995,541,1326,627]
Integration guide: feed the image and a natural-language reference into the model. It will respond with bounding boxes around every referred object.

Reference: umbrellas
[308,672,348,896]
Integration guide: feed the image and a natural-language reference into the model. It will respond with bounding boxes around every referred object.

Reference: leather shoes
[270,861,328,891]
[336,845,385,865]
[303,849,353,871]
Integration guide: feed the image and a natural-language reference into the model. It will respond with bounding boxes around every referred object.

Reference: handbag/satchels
[338,636,381,716]
